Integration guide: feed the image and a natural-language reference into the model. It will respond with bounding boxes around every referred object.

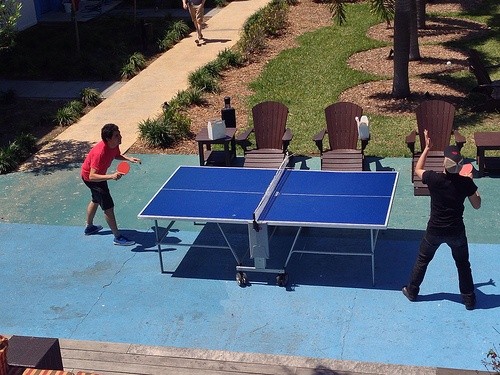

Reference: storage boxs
[207,120,227,140]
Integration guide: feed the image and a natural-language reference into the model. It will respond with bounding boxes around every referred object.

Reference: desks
[5,335,64,375]
[473,131,500,178]
[195,128,238,167]
[135,165,399,288]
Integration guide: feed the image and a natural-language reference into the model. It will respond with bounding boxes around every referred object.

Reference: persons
[402,129,482,310]
[80,123,140,246]
[183,0,206,43]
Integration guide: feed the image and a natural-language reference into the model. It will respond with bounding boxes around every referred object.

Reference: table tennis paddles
[458,164,473,176]
[116,162,130,175]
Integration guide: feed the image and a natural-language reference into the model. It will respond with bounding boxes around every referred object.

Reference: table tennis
[446,60,451,66]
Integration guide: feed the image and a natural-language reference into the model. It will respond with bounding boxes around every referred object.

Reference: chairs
[235,101,294,168]
[311,101,371,171]
[404,99,467,196]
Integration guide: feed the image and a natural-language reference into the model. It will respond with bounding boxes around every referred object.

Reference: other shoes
[402,285,416,302]
[113,235,135,246]
[195,39,199,45]
[84,224,103,235]
[465,298,475,310]
[198,34,203,39]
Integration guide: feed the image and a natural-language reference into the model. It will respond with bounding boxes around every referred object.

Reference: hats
[444,145,464,174]
